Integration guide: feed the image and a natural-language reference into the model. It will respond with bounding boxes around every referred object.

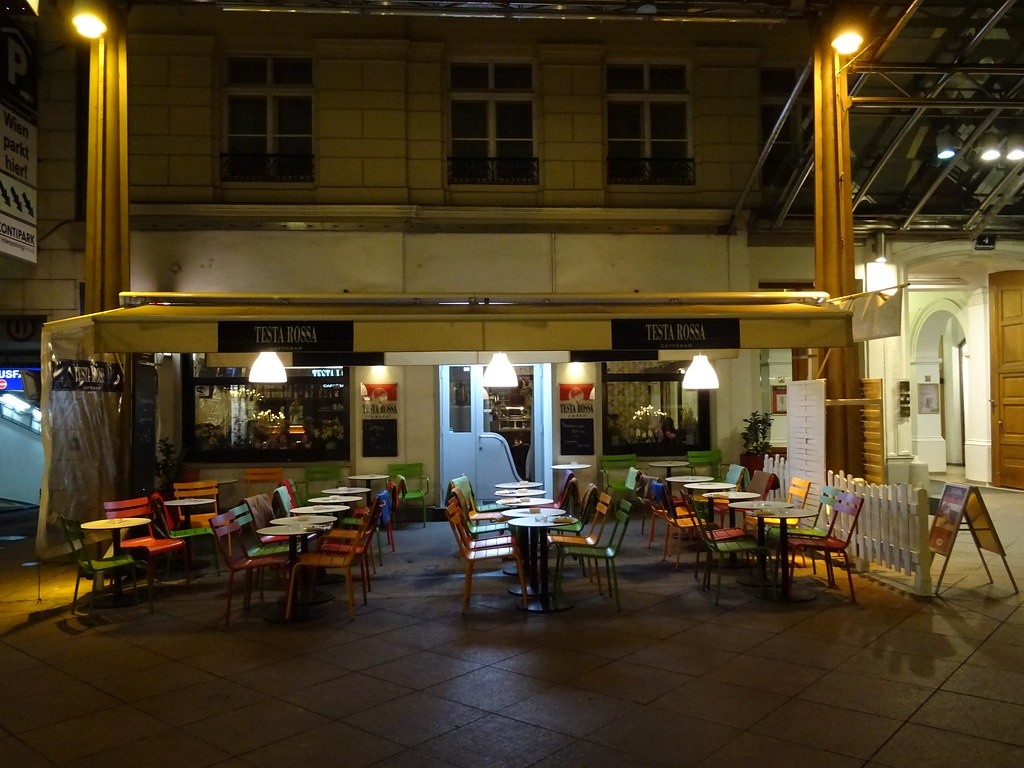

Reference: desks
[163,499,217,570]
[551,464,591,516]
[257,476,389,623]
[745,509,819,603]
[665,476,714,540]
[495,481,579,613]
[216,479,237,514]
[728,500,794,586]
[648,461,690,495]
[684,482,737,553]
[80,518,152,608]
[702,492,761,568]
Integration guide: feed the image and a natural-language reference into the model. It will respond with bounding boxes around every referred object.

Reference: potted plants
[739,410,776,482]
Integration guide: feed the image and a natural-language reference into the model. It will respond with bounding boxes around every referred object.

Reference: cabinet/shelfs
[247,377,349,453]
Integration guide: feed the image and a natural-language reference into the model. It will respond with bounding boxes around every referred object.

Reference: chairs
[60,460,342,627]
[600,449,865,605]
[286,474,408,622]
[536,470,633,616]
[444,474,528,616]
[387,463,430,529]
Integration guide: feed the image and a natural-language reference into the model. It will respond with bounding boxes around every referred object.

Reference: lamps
[682,349,719,390]
[830,9,863,53]
[482,351,518,388]
[872,230,887,263]
[1007,134,1024,160]
[249,352,287,383]
[72,1,107,39]
[936,132,955,159]
[980,131,999,161]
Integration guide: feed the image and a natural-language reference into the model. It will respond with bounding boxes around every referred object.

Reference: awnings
[91,304,854,353]
